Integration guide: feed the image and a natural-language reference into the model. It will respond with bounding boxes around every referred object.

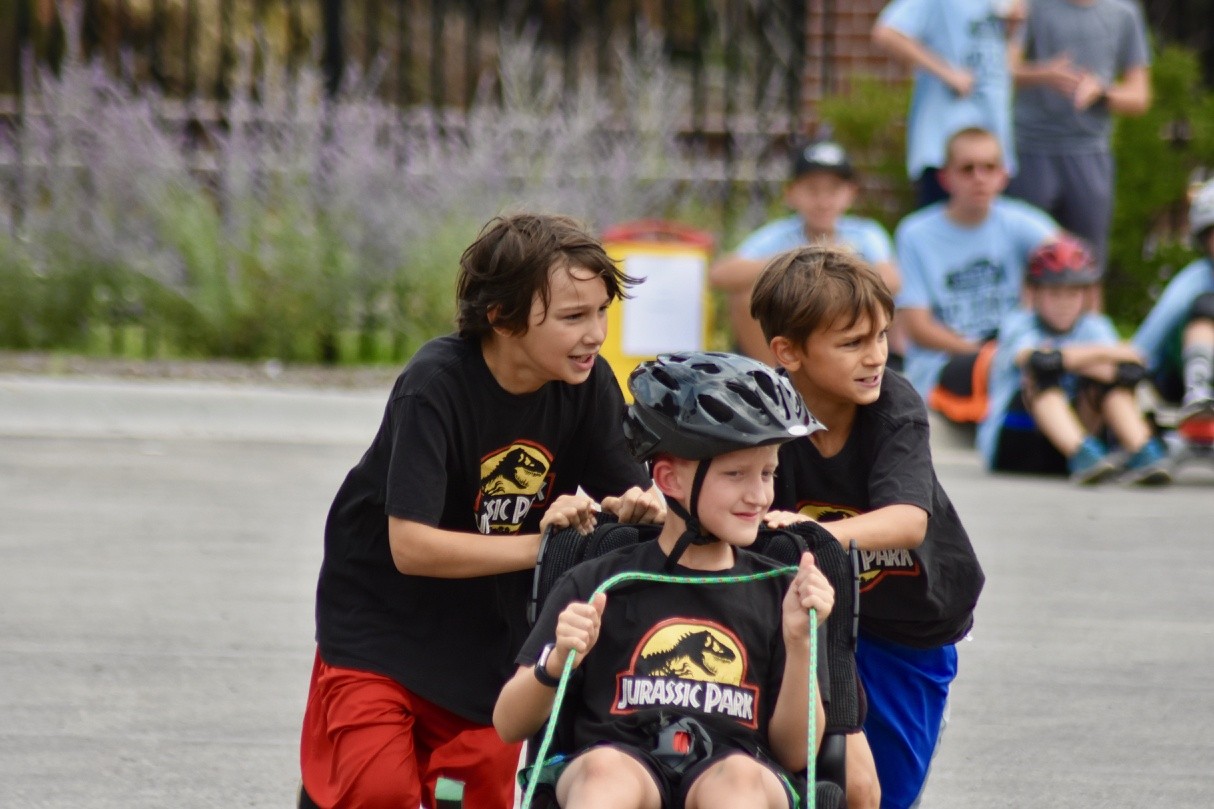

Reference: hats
[793,140,853,178]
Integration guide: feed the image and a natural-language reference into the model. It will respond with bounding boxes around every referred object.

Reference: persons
[300,215,670,809]
[711,138,902,370]
[867,1,1029,207]
[893,126,1070,442]
[492,350,834,809]
[1132,178,1214,449]
[1005,1,1156,310]
[976,232,1179,487]
[749,247,986,809]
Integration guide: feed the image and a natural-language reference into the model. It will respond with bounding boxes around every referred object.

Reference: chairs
[523,514,858,809]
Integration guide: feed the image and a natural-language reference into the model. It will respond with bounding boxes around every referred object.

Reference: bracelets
[1089,92,1108,111]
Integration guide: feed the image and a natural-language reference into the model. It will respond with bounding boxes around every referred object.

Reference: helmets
[1027,241,1102,286]
[1189,179,1214,238]
[621,352,829,464]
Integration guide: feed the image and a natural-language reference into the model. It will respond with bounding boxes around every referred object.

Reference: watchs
[534,644,560,688]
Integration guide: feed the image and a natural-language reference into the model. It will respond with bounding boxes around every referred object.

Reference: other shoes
[1079,458,1119,485]
[1133,466,1172,486]
